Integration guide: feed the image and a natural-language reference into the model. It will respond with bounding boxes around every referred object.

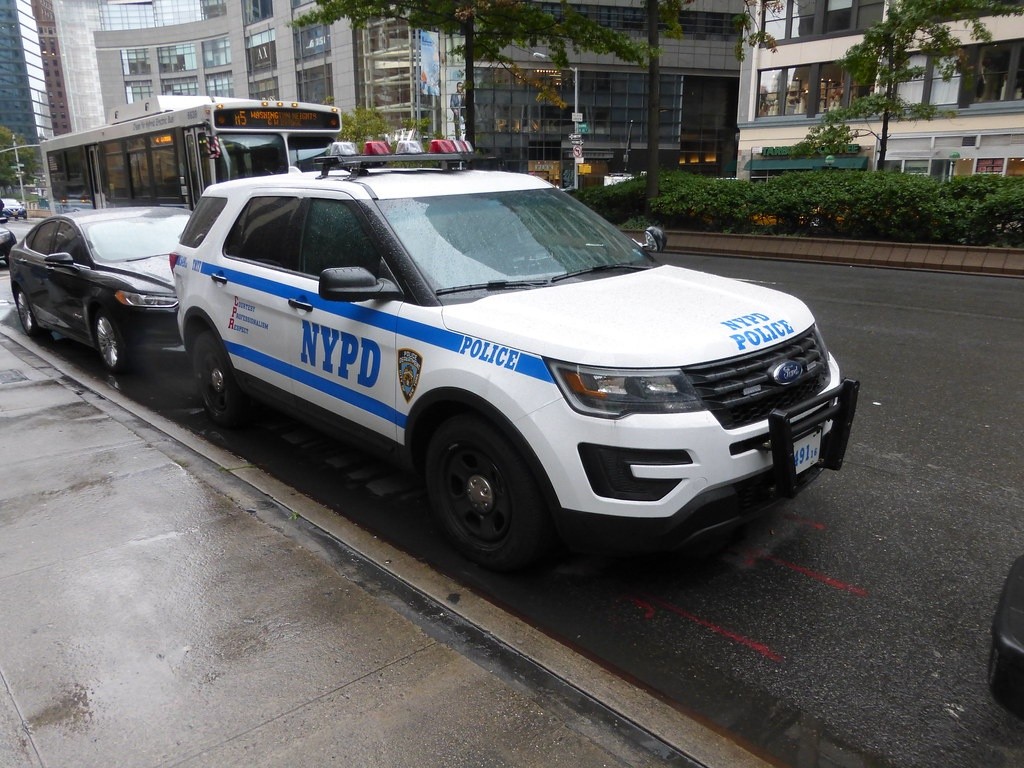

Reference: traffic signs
[568,134,584,146]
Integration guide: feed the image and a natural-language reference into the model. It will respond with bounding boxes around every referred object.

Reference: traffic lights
[577,164,592,175]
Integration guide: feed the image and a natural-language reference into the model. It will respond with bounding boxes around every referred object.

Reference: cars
[0,229,16,269]
[9,208,199,375]
[1,198,29,219]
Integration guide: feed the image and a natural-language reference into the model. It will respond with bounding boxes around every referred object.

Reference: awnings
[743,155,869,171]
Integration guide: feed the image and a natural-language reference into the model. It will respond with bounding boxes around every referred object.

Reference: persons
[450,82,468,141]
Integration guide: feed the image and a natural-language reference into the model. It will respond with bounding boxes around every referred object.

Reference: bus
[39,95,341,217]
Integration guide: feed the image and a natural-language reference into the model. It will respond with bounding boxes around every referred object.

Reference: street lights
[11,134,25,200]
[533,52,578,191]
[947,151,960,183]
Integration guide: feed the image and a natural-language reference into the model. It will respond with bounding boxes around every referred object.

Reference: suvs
[166,141,865,557]
